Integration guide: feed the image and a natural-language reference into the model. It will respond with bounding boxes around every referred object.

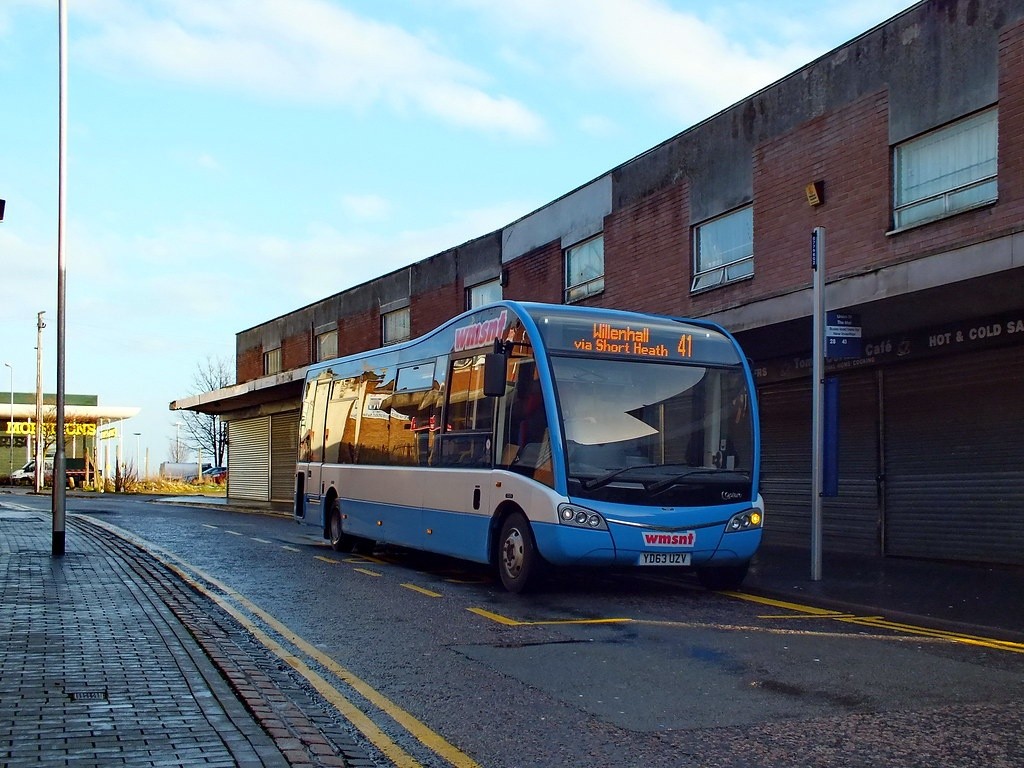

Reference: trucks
[158,461,213,482]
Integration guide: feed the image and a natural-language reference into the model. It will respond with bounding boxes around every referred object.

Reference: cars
[187,466,227,486]
[10,456,102,488]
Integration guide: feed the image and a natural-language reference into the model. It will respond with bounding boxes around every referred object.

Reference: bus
[293,298,767,595]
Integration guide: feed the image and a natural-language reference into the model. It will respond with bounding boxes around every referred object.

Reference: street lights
[172,423,180,463]
[133,432,141,482]
[5,363,14,473]
